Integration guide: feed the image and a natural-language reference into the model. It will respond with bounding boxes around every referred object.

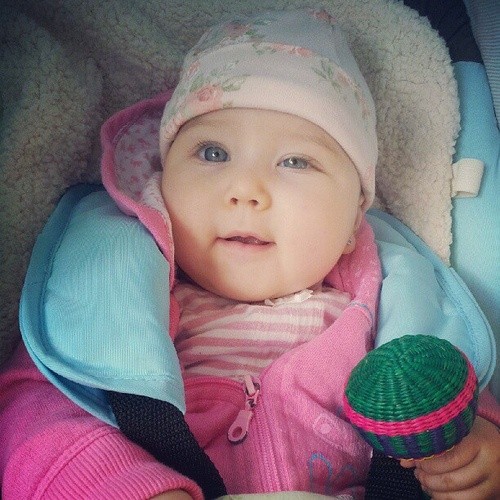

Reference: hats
[160,9,378,211]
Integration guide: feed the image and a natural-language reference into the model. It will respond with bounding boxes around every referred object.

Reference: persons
[3,7,499,499]
